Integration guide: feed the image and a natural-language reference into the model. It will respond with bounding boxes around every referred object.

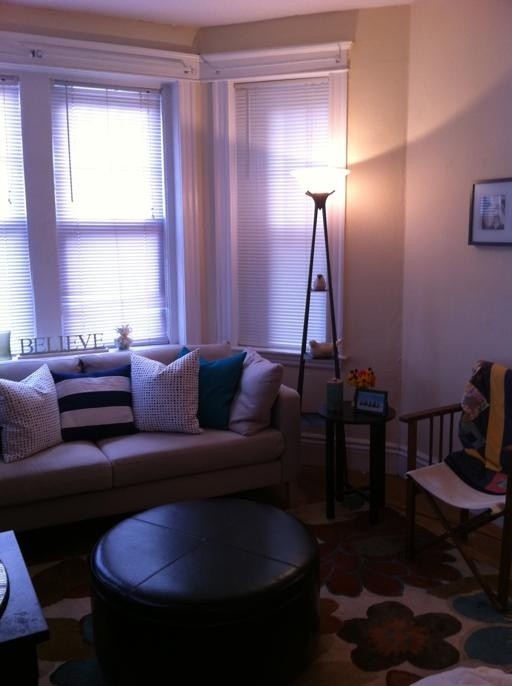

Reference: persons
[359,399,379,408]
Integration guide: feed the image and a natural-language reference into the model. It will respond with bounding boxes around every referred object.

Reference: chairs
[385,362,510,617]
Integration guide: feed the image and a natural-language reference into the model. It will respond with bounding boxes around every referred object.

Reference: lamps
[282,159,357,518]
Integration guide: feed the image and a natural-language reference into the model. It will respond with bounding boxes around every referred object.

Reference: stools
[80,496,326,684]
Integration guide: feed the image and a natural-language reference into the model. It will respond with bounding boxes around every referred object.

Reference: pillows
[176,339,250,432]
[0,360,66,466]
[226,345,287,437]
[127,345,207,439]
[49,361,140,444]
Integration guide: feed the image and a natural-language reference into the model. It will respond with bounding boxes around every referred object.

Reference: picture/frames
[353,385,389,416]
[467,176,512,247]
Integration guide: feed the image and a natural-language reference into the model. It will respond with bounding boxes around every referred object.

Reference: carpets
[15,486,512,685]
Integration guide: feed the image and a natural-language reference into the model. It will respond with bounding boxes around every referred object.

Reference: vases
[312,273,327,290]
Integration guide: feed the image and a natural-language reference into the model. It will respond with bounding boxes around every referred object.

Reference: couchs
[0,337,313,536]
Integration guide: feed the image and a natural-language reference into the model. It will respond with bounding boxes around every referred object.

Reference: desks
[1,523,53,686]
[309,393,401,519]
